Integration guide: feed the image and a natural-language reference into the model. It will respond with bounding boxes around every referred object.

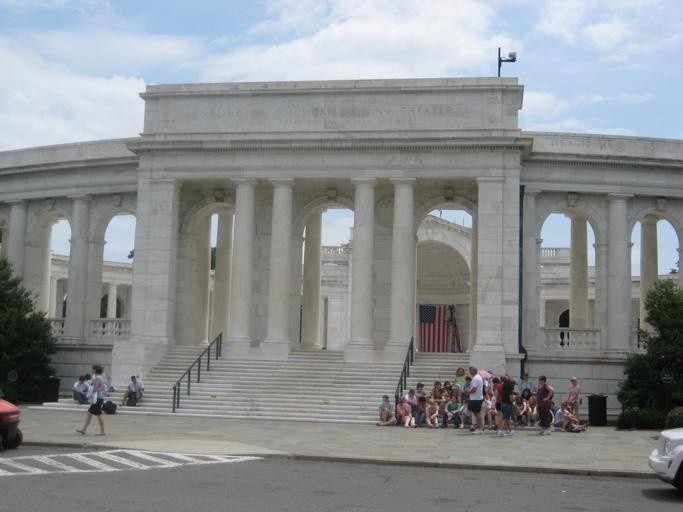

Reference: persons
[122,375,144,407]
[375,366,587,435]
[71,365,108,435]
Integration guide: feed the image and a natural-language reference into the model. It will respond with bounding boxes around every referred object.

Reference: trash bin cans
[588,396,608,425]
[46,376,59,402]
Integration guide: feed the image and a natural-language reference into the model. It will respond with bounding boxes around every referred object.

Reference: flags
[418,303,454,352]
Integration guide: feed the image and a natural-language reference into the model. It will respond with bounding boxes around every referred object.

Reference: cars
[650,428,683,491]
[0,399,22,449]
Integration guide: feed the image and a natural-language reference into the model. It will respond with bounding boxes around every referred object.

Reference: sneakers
[497,431,512,436]
[459,424,484,435]
